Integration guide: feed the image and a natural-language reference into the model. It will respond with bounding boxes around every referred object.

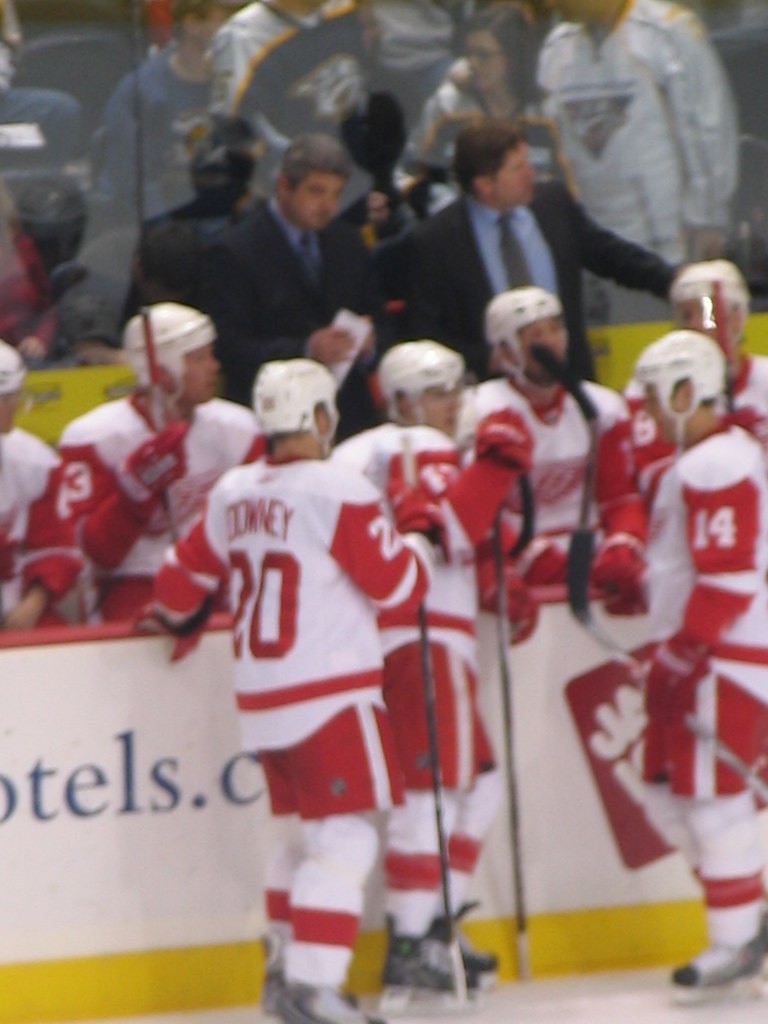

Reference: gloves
[595,544,652,619]
[387,464,449,534]
[647,633,710,714]
[474,407,537,473]
[118,421,190,511]
[483,577,539,648]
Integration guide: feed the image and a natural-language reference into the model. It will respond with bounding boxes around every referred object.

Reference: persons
[152,360,451,1024]
[331,340,537,992]
[0,0,768,630]
[633,330,768,989]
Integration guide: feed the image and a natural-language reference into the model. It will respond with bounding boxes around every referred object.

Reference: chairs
[14,25,136,140]
[74,231,140,340]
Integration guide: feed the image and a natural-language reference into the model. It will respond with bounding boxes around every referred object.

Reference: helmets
[124,303,217,387]
[486,286,563,343]
[637,330,727,418]
[253,360,340,436]
[672,258,750,307]
[1,340,27,394]
[378,340,465,400]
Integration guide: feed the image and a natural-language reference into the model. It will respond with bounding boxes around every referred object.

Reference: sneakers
[672,923,768,1008]
[378,902,499,1015]
[259,930,387,1024]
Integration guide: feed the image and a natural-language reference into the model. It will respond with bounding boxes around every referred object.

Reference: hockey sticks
[712,280,734,414]
[414,601,468,1010]
[534,345,766,805]
[140,308,177,540]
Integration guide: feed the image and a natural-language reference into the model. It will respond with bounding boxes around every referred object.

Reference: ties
[497,215,533,288]
[296,232,318,289]
